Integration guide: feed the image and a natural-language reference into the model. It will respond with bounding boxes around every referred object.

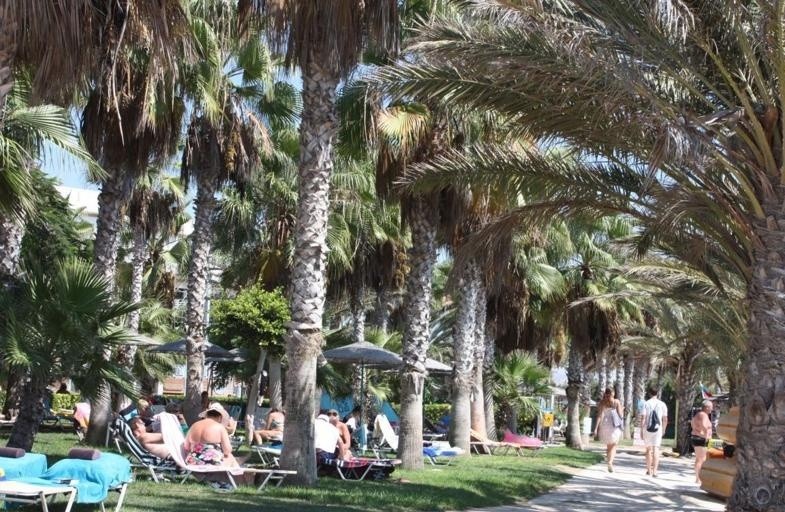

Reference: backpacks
[647,408,662,433]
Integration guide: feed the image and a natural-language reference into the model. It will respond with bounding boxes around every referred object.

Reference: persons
[126,390,286,475]
[690,399,713,484]
[314,405,361,461]
[54,383,70,393]
[640,385,668,477]
[40,387,58,418]
[434,408,453,433]
[592,386,625,474]
[70,408,85,441]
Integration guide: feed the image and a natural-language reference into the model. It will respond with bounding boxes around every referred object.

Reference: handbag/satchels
[611,407,623,428]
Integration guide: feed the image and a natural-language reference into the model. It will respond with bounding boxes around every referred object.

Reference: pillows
[0,447,26,458]
[69,448,101,460]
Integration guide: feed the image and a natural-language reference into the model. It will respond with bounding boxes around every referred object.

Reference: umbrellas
[382,356,453,376]
[321,341,403,424]
[145,335,241,361]
[73,402,91,431]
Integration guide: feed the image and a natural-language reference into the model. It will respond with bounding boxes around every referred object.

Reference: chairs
[0,452,134,512]
[105,403,546,490]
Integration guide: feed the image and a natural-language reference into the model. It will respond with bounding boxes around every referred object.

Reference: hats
[198,402,231,422]
